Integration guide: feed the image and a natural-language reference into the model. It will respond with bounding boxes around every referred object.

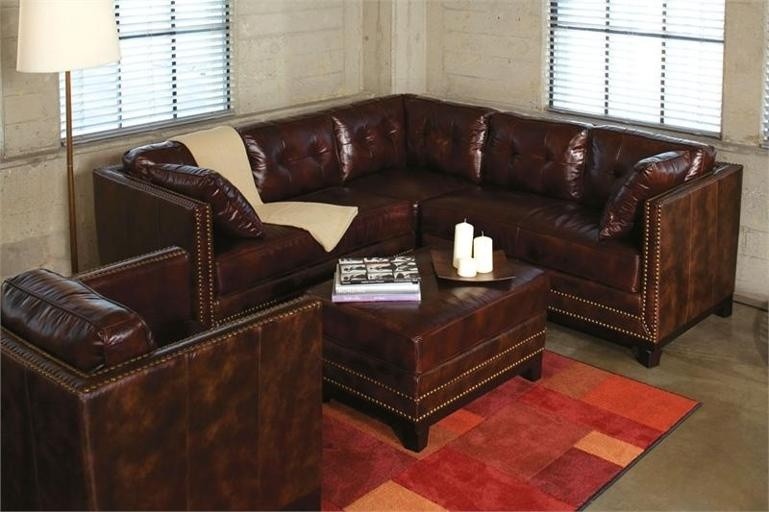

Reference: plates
[428,248,517,284]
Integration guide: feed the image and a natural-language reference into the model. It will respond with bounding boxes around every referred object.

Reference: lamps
[15,1,123,274]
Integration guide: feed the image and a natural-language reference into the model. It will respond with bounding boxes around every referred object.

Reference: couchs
[92,95,744,369]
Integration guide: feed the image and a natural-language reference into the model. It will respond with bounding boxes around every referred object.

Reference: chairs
[0,244,324,511]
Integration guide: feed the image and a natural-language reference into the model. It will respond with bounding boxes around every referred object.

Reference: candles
[453,216,493,277]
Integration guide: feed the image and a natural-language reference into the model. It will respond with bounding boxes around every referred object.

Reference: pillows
[0,266,155,374]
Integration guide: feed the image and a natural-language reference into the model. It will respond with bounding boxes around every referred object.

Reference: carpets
[323,349,702,512]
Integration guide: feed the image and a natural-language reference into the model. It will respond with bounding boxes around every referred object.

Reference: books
[331,255,421,303]
